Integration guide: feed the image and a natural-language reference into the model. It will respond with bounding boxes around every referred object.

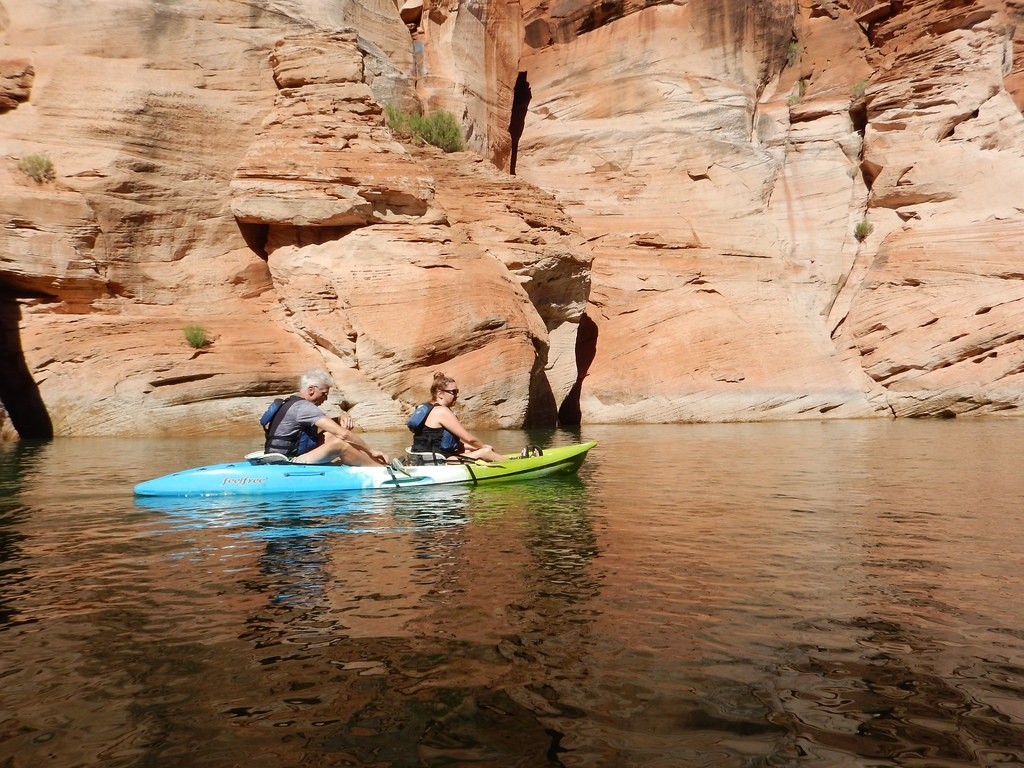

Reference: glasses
[441,389,458,395]
[314,386,328,398]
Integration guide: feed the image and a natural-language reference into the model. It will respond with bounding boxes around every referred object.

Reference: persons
[264,366,391,468]
[411,371,543,462]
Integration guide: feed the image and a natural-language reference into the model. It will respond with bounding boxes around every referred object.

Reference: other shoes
[521,444,529,458]
[530,446,543,458]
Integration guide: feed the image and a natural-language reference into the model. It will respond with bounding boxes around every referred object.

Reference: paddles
[389,456,410,478]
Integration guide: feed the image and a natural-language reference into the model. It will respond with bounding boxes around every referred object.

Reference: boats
[133,440,597,497]
